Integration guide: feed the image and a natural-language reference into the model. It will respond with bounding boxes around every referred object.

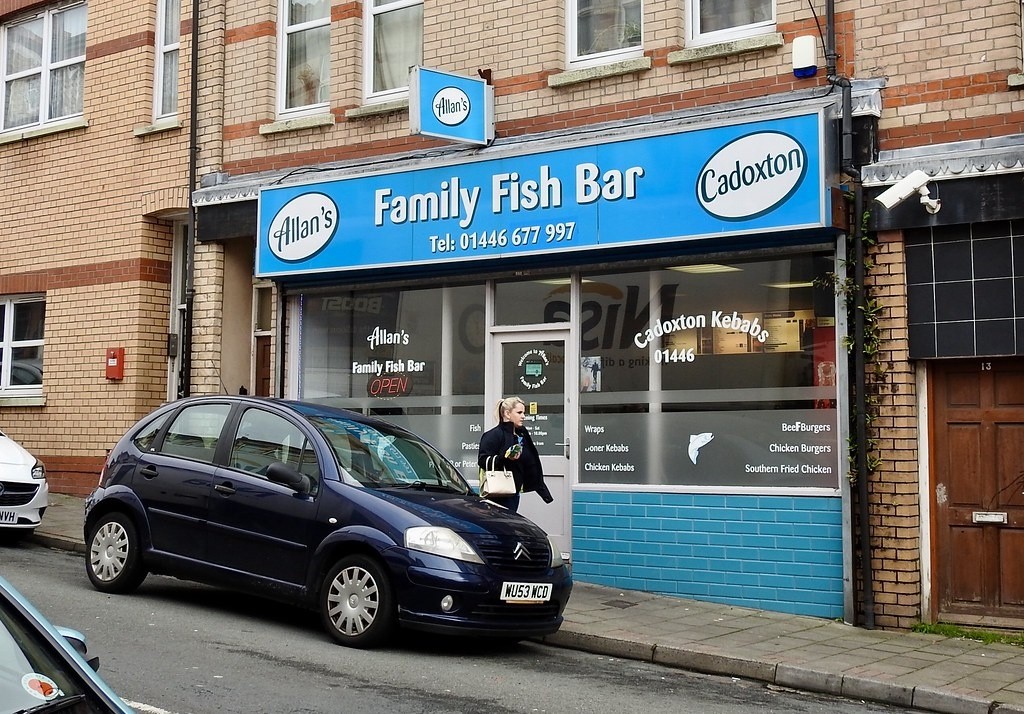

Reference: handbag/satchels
[478,455,516,497]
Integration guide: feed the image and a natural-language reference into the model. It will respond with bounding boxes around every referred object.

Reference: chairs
[174,432,391,490]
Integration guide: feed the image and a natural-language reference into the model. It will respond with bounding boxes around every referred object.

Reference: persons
[477,397,554,512]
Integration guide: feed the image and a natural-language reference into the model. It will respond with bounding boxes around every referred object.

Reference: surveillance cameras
[875,170,941,214]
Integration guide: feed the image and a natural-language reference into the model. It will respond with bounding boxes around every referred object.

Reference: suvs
[81,396,574,652]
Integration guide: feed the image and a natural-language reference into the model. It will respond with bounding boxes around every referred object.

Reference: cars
[0,428,49,538]
[0,358,43,385]
[0,576,133,714]
